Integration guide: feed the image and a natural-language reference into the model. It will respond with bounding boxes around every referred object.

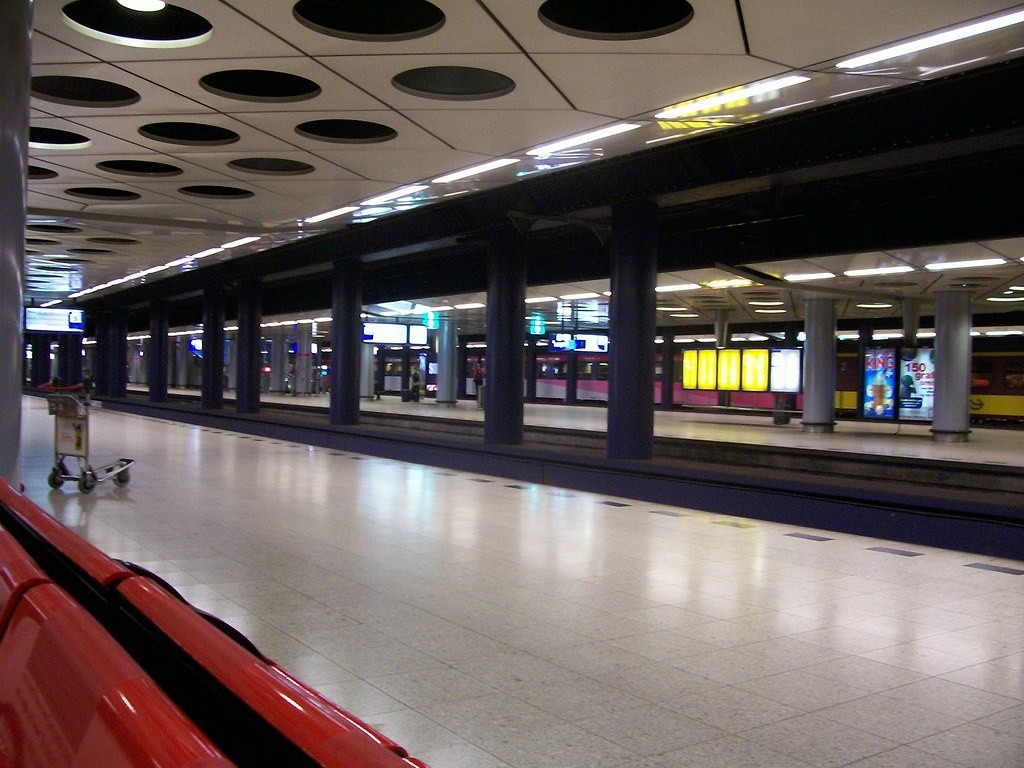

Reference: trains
[313,331,1024,425]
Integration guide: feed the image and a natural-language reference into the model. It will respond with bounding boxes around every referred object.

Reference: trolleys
[37,378,136,494]
